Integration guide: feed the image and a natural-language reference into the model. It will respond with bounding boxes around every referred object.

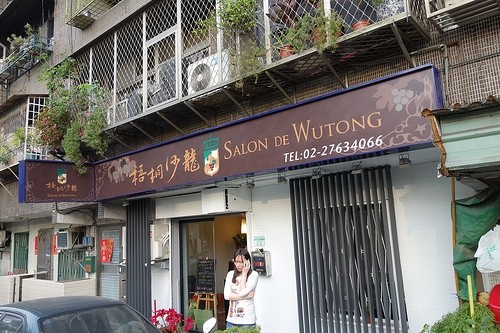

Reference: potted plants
[266,0,404,59]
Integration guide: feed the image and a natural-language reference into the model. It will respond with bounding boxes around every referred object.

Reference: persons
[224,249,259,329]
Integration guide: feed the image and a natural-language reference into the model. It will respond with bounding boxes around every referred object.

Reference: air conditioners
[188,52,233,106]
[56,230,85,249]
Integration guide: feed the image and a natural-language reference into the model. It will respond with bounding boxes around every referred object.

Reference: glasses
[234,262,243,266]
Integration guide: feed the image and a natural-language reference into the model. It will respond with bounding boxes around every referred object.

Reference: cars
[0,295,164,333]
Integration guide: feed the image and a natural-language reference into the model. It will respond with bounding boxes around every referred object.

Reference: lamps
[246,179,255,188]
[311,167,321,179]
[399,153,411,168]
[351,162,363,174]
[277,173,287,183]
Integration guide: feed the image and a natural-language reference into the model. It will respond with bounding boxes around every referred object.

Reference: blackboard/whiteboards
[197,259,216,293]
